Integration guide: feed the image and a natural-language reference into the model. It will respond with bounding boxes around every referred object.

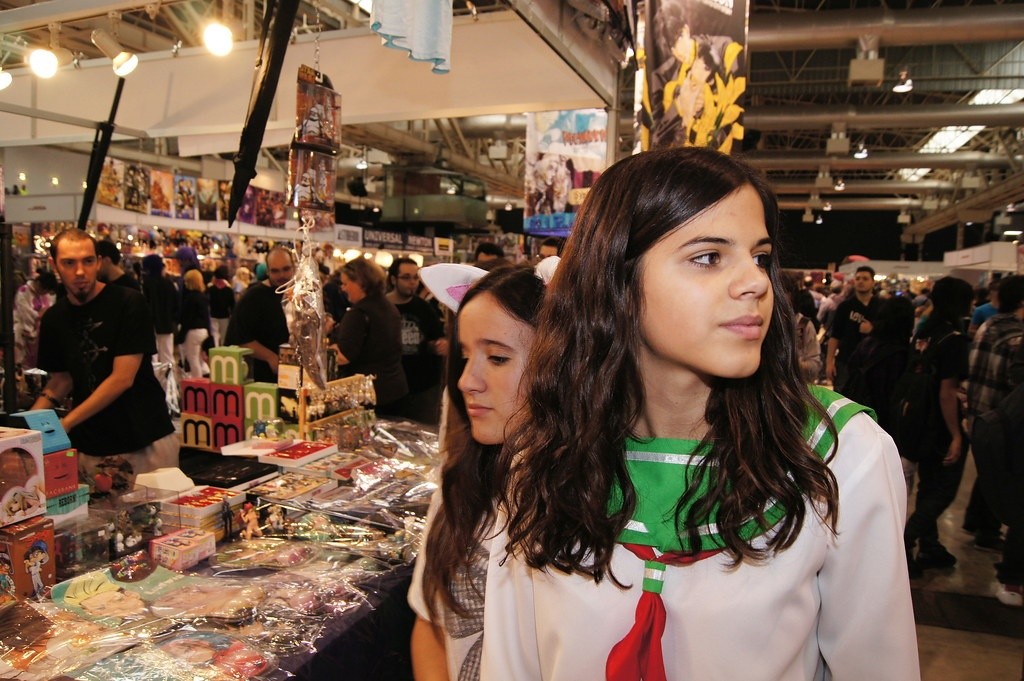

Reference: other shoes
[917,540,955,568]
[904,544,922,578]
[906,475,914,495]
[961,521,1003,536]
[994,581,1023,608]
[974,535,1004,555]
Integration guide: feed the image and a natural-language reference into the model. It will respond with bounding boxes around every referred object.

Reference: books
[122,437,339,545]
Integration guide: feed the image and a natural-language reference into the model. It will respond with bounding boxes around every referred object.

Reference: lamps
[802,191,832,225]
[205,15,246,55]
[825,122,850,156]
[28,21,74,78]
[892,66,913,94]
[487,129,512,161]
[897,209,911,224]
[0,63,13,90]
[853,141,869,160]
[815,164,833,189]
[91,10,139,77]
[846,34,885,88]
[834,177,846,191]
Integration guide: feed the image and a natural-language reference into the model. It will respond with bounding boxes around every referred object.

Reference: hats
[165,247,197,262]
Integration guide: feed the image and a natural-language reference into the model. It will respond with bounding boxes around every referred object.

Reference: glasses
[397,274,419,281]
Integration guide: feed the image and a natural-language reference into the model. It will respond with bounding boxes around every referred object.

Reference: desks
[266,558,418,681]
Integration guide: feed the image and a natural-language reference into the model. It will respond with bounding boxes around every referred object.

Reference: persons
[782,265,1024,606]
[0,227,569,493]
[406,265,549,681]
[480,148,921,681]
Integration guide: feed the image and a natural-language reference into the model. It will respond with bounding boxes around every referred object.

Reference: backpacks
[839,339,910,408]
[973,384,1024,523]
[890,330,963,463]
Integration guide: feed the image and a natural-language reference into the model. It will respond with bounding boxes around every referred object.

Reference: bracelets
[39,393,60,408]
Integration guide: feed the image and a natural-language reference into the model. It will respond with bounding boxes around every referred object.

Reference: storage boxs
[0,345,375,604]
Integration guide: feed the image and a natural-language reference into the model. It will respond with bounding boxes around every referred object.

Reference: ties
[605,540,731,680]
